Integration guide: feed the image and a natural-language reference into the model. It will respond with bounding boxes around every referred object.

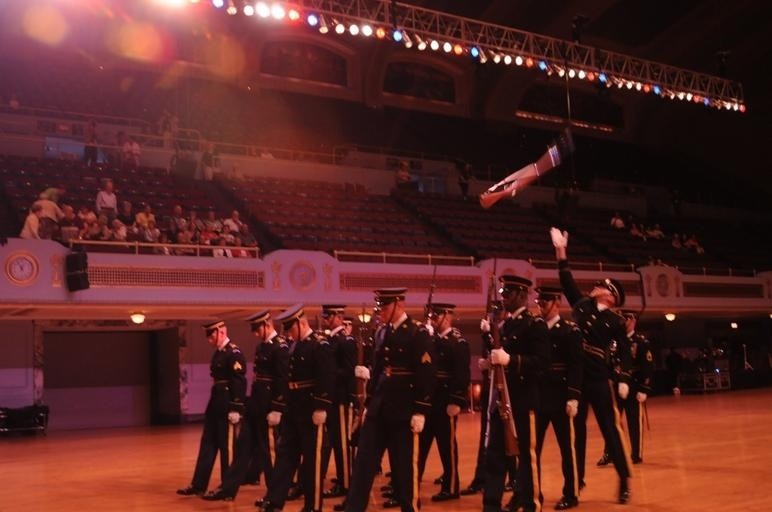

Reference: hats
[373,287,407,306]
[243,304,353,333]
[594,277,625,307]
[488,275,562,312]
[427,302,456,318]
[202,320,224,336]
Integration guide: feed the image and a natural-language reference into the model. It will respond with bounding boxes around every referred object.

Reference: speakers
[68,272,90,291]
[66,253,87,273]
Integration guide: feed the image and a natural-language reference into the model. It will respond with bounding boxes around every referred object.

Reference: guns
[480,146,561,207]
[481,255,522,457]
[354,304,368,440]
[421,264,437,329]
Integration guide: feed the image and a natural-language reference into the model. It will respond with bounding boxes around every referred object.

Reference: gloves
[267,412,281,426]
[480,318,490,331]
[619,382,628,398]
[227,413,240,423]
[355,366,371,379]
[448,405,460,417]
[638,393,647,403]
[412,415,424,432]
[566,399,578,417]
[551,227,568,249]
[478,349,510,369]
[312,411,327,426]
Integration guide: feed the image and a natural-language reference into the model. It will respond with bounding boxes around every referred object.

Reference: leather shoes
[255,478,345,512]
[556,497,578,509]
[380,469,398,509]
[432,475,479,500]
[177,486,235,500]
[505,480,516,492]
[597,453,611,466]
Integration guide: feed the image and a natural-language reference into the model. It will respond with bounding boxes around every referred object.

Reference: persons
[122,135,142,169]
[172,143,216,184]
[473,274,556,511]
[503,283,584,511]
[394,161,421,193]
[21,179,257,259]
[460,297,514,496]
[598,212,718,269]
[456,161,474,195]
[597,305,646,467]
[550,226,636,510]
[158,106,179,150]
[83,119,103,166]
[173,282,473,510]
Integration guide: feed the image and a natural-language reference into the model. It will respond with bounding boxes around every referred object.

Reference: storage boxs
[679,353,733,393]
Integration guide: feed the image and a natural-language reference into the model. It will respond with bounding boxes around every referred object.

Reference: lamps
[128,312,147,325]
[356,311,374,324]
[211,0,748,115]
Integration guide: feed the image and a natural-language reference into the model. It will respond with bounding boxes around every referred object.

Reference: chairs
[0,152,771,273]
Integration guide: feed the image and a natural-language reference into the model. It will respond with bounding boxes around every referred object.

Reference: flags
[481,360,522,459]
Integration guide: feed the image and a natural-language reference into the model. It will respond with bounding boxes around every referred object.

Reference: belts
[254,374,270,381]
[289,379,311,389]
[583,343,606,359]
[383,365,411,376]
[437,371,448,376]
